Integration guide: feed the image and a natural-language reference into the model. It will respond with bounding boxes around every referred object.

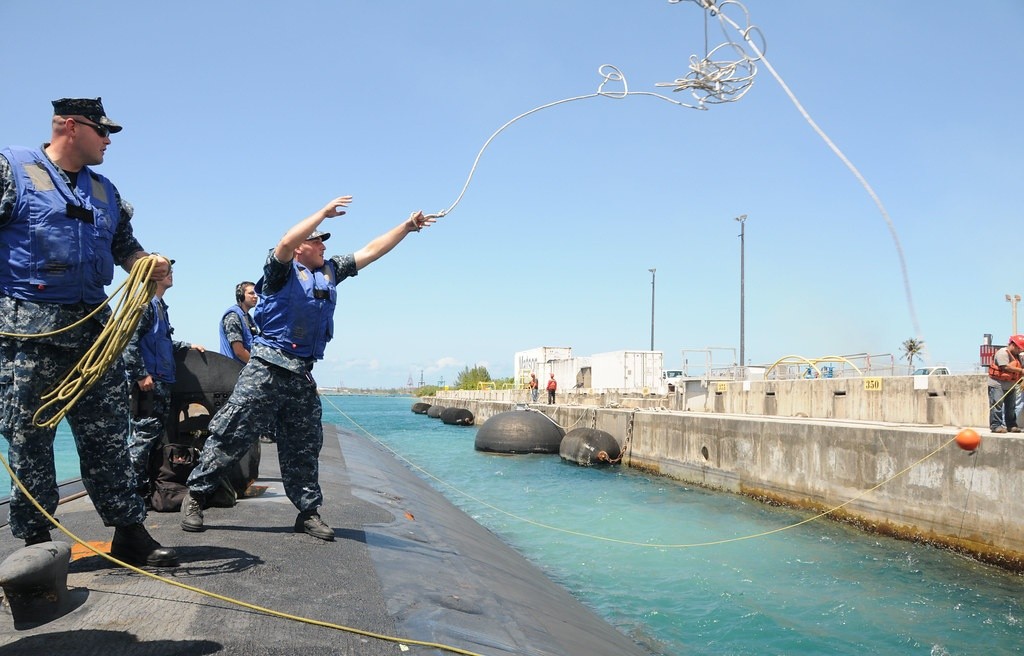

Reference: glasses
[75,120,110,137]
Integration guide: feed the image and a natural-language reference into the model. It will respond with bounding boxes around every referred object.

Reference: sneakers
[111,521,180,567]
[180,490,205,533]
[293,512,335,540]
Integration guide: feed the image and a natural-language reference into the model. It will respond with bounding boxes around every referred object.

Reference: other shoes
[994,425,1007,433]
[1009,427,1021,432]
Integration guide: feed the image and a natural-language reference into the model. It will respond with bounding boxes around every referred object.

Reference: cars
[663,370,687,385]
[911,367,951,375]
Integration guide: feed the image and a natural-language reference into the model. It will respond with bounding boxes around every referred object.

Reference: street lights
[649,268,657,351]
[1005,294,1020,336]
[734,214,747,377]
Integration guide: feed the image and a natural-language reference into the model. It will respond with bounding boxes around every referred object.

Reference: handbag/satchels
[146,440,239,513]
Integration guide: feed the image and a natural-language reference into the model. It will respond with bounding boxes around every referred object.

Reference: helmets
[1010,335,1024,350]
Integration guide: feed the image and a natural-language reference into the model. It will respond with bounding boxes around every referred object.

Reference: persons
[180,195,437,536]
[219,282,261,497]
[531,373,538,402]
[0,96,179,566]
[547,374,557,404]
[121,252,204,499]
[988,335,1024,433]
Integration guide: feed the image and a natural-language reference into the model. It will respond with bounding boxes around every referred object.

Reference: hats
[152,251,176,266]
[51,97,122,134]
[306,229,332,242]
